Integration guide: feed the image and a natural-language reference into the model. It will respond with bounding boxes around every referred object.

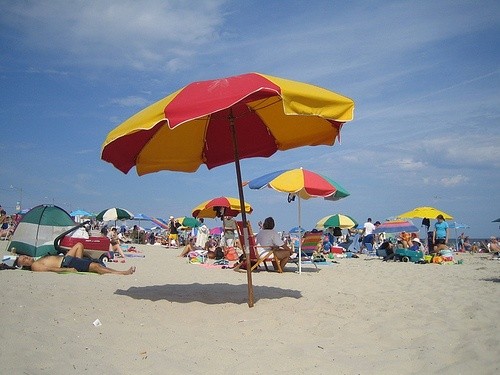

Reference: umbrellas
[192,197,253,236]
[100,74,354,307]
[289,227,305,238]
[168,216,199,232]
[448,220,472,251]
[134,215,152,224]
[315,213,355,242]
[210,227,223,239]
[148,218,168,229]
[88,214,99,220]
[80,212,94,223]
[242,166,351,275]
[356,224,365,233]
[396,207,453,253]
[15,208,33,215]
[492,217,500,229]
[69,210,92,222]
[96,208,134,227]
[373,216,420,241]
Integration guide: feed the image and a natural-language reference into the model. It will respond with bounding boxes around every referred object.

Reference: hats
[412,238,421,246]
[169,216,174,221]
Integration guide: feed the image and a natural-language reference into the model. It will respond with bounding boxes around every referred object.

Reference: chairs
[288,230,323,273]
[233,220,283,273]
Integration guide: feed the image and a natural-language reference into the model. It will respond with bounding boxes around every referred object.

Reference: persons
[311,229,318,232]
[0,205,22,241]
[195,217,209,248]
[111,244,129,257]
[255,217,294,272]
[356,218,375,253]
[13,241,136,275]
[472,238,490,254]
[376,232,422,261]
[178,240,224,260]
[374,221,382,228]
[107,225,156,244]
[168,216,180,249]
[490,237,500,254]
[433,215,449,246]
[258,221,263,229]
[458,233,472,252]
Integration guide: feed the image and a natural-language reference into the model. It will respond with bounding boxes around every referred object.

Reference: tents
[5,204,77,257]
